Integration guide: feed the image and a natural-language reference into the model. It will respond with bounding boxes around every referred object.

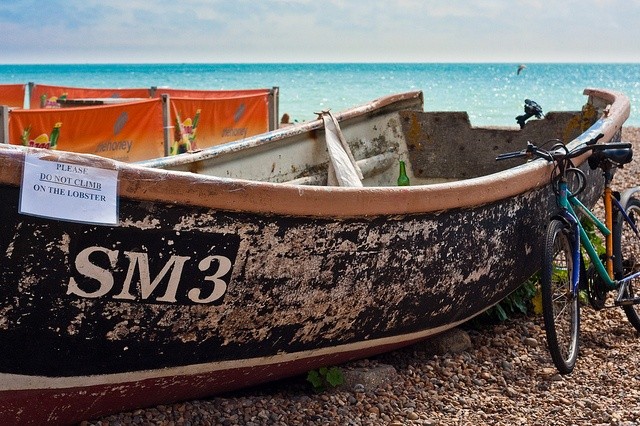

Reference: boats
[0,86,630,424]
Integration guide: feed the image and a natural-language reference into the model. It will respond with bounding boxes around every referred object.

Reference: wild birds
[524,98,544,120]
[517,64,527,76]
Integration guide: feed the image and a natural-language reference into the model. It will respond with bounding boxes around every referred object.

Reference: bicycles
[495,133,639,375]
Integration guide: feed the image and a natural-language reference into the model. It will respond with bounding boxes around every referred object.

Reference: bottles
[396,160,410,186]
